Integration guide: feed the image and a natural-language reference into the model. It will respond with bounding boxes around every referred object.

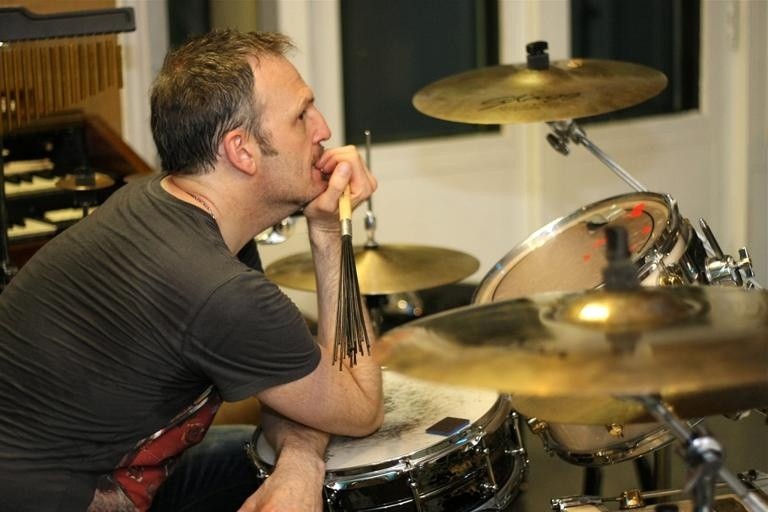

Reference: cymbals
[266,246,481,298]
[409,54,664,131]
[510,397,768,427]
[369,286,768,396]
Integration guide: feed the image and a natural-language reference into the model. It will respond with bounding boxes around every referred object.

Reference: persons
[1,26,385,512]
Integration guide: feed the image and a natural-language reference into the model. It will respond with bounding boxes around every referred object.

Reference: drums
[245,368,528,512]
[474,189,714,466]
